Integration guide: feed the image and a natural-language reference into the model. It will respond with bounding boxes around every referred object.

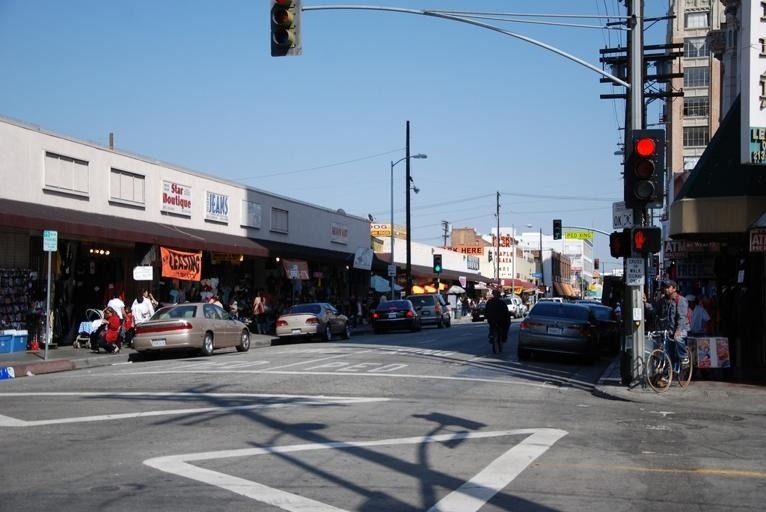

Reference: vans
[537,297,562,303]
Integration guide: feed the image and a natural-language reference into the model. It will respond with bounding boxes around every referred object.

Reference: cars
[577,301,618,357]
[371,300,421,333]
[275,303,350,341]
[473,298,527,321]
[517,300,601,364]
[407,295,451,329]
[133,303,250,355]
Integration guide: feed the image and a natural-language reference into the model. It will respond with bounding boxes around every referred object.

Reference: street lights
[512,222,532,293]
[391,154,428,300]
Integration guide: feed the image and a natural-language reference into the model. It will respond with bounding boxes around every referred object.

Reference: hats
[663,279,677,287]
[685,295,696,301]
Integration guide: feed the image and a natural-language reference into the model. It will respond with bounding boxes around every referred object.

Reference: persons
[656,278,756,381]
[205,293,224,308]
[252,290,268,335]
[483,289,512,344]
[228,295,238,319]
[99,285,179,353]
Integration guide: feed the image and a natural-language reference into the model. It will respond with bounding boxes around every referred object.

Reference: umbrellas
[371,274,403,292]
[412,281,466,298]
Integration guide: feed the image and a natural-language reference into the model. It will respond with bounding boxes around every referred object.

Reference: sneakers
[681,356,690,365]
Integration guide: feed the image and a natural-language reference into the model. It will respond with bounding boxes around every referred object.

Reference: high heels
[111,348,120,355]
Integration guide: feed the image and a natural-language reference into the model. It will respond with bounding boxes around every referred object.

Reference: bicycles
[648,330,693,391]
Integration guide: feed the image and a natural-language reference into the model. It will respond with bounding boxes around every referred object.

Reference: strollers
[73,307,104,349]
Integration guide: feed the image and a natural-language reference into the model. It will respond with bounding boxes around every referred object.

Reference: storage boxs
[14,330,28,352]
[0,329,15,353]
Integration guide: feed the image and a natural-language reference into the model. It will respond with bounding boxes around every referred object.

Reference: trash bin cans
[0,329,29,353]
[455,310,462,319]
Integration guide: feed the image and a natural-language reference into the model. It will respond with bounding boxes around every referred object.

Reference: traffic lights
[553,219,562,240]
[271,0,302,55]
[630,226,661,254]
[625,128,663,208]
[433,254,442,273]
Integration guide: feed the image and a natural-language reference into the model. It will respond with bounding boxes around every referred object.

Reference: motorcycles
[491,329,503,353]
